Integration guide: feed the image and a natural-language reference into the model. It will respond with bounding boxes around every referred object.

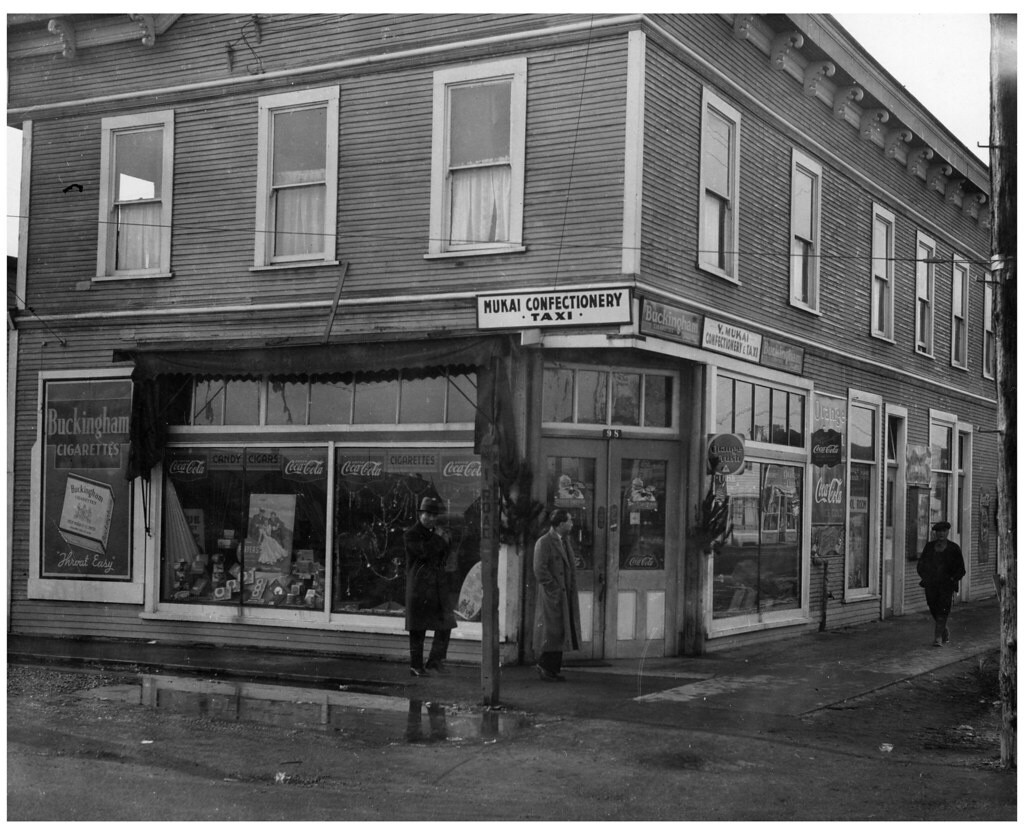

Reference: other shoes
[426,658,451,675]
[536,664,565,682]
[410,665,429,676]
[932,639,943,647]
[943,631,949,643]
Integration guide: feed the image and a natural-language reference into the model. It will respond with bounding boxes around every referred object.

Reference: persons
[404,496,458,678]
[533,510,584,681]
[251,507,289,565]
[916,521,966,646]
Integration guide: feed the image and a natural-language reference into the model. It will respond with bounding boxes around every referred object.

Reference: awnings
[111,328,521,388]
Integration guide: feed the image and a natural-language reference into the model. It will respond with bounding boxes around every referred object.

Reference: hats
[932,521,952,531]
[417,496,446,514]
